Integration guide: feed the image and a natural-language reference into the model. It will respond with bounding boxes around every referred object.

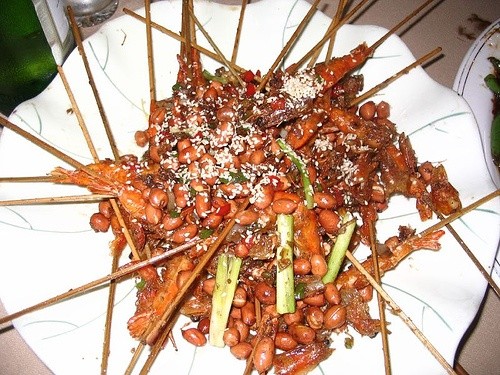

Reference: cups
[60,0,119,28]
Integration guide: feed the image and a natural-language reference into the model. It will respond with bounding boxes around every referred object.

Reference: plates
[451,19,500,298]
[0,0,500,375]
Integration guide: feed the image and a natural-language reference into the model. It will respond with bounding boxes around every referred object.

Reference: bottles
[0,0,80,118]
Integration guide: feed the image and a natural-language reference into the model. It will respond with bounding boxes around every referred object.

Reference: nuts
[89,81,435,374]
